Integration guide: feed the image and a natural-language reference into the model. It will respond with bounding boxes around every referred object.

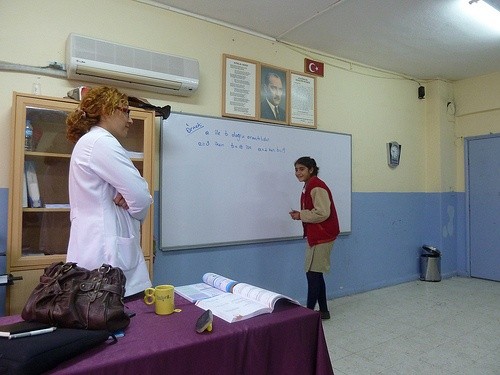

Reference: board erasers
[195,309,213,334]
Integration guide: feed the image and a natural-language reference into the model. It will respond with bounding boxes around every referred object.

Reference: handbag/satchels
[0,321,116,375]
[19,259,129,335]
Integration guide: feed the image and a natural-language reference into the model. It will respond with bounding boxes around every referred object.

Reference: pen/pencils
[10,325,58,335]
[9,327,56,341]
[129,313,137,318]
[108,333,126,339]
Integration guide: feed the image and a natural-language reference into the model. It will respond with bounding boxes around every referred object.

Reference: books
[194,282,302,324]
[173,272,240,304]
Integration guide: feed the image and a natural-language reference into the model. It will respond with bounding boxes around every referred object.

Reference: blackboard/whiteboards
[159,110,353,252]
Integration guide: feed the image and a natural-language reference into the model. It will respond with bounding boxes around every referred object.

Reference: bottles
[24,120,33,150]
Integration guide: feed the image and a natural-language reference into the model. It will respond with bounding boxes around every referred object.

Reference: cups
[144,285,175,316]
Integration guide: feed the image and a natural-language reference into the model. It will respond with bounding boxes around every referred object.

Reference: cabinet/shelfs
[6,91,155,317]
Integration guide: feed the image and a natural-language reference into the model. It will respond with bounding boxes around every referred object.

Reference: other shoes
[317,310,330,318]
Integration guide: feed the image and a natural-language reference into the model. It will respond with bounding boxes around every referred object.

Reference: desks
[0,290,333,375]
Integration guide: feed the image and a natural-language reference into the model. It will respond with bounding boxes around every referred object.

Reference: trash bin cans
[419,245,441,282]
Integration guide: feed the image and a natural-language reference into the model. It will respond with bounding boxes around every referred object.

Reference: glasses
[274,106,279,121]
[113,106,130,117]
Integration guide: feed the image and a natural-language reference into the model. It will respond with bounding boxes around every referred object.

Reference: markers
[291,207,295,211]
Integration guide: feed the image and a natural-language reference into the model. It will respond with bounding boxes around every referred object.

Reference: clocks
[387,142,401,168]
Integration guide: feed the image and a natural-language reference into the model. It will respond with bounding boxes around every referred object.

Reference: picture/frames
[222,53,259,121]
[259,62,289,126]
[290,69,318,128]
[305,58,325,78]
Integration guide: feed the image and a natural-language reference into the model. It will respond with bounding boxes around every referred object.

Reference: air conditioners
[65,33,199,97]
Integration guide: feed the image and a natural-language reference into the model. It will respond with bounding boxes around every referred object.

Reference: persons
[261,71,285,121]
[67,85,153,302]
[290,157,341,320]
